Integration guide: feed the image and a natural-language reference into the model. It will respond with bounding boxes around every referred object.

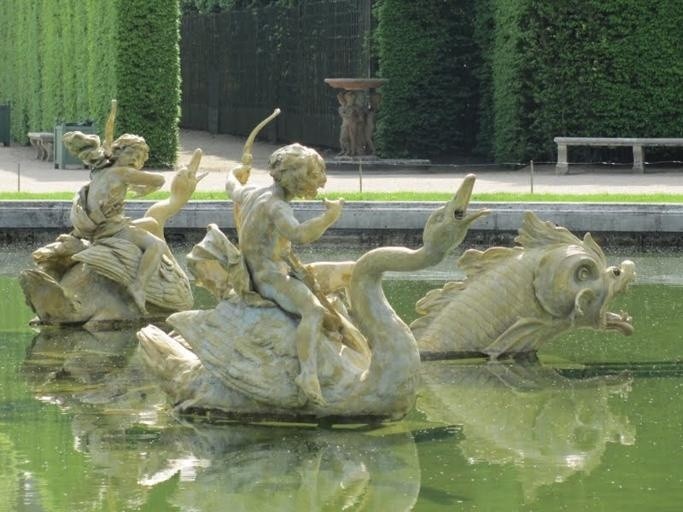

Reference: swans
[17,146,212,326]
[125,171,495,416]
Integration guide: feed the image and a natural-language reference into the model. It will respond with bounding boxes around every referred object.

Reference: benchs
[27,132,54,162]
[554,136,683,173]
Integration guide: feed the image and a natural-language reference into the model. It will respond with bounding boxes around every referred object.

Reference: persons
[68,132,167,315]
[336,90,381,157]
[224,141,357,409]
